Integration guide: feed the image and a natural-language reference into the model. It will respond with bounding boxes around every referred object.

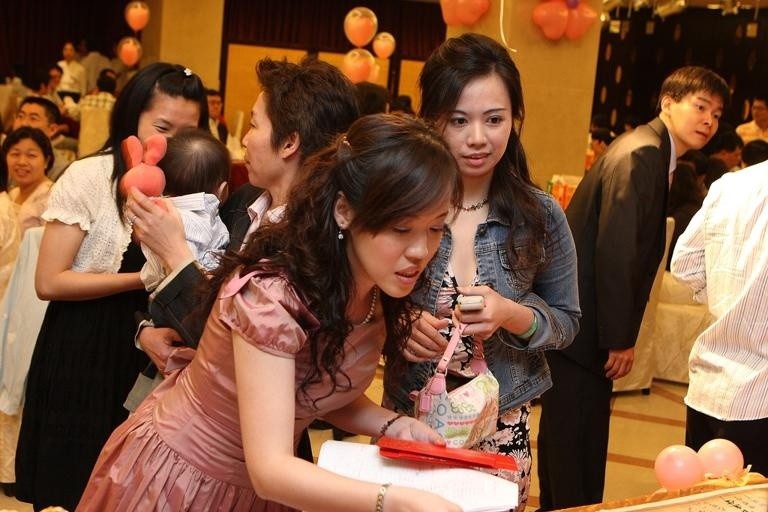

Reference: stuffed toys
[119,134,168,212]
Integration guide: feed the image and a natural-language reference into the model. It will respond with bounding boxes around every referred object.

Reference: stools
[646,304,717,384]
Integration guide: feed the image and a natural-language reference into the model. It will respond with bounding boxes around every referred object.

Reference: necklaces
[359,286,379,327]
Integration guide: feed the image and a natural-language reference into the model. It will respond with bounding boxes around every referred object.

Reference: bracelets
[516,310,537,338]
[374,481,391,511]
[380,413,407,438]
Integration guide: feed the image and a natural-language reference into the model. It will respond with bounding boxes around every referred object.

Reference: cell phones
[458,294,484,313]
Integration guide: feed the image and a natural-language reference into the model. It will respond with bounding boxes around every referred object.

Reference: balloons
[654,443,699,489]
[436,0,603,42]
[696,437,744,477]
[342,6,376,47]
[372,34,394,59]
[124,1,149,33]
[346,49,377,81]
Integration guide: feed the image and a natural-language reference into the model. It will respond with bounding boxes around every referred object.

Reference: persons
[203,87,228,144]
[667,154,767,483]
[14,60,216,512]
[379,32,580,511]
[668,89,767,271]
[124,126,233,407]
[588,116,625,154]
[533,62,729,507]
[1,33,229,296]
[122,56,366,420]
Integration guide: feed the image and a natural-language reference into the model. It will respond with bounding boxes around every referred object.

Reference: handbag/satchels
[409,323,500,449]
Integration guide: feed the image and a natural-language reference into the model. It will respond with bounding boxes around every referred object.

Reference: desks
[226,155,249,193]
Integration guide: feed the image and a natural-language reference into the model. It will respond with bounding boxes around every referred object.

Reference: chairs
[1,226,53,426]
[612,216,675,399]
[75,107,112,156]
[225,110,244,140]
